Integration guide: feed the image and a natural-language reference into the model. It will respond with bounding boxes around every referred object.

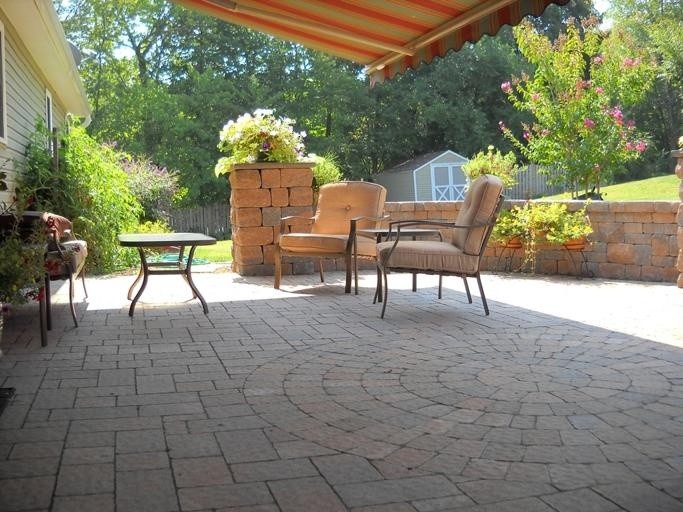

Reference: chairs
[373,173,505,319]
[0,210,88,327]
[274,181,387,295]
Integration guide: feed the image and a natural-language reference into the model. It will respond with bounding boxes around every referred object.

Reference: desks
[19,244,52,347]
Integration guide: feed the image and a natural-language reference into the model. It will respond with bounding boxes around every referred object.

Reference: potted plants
[490,198,596,280]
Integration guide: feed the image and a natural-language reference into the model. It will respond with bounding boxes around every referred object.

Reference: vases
[257,151,274,163]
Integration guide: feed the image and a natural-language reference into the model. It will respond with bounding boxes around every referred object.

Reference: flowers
[219,107,307,164]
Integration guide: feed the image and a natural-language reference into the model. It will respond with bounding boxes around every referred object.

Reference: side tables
[357,229,444,304]
